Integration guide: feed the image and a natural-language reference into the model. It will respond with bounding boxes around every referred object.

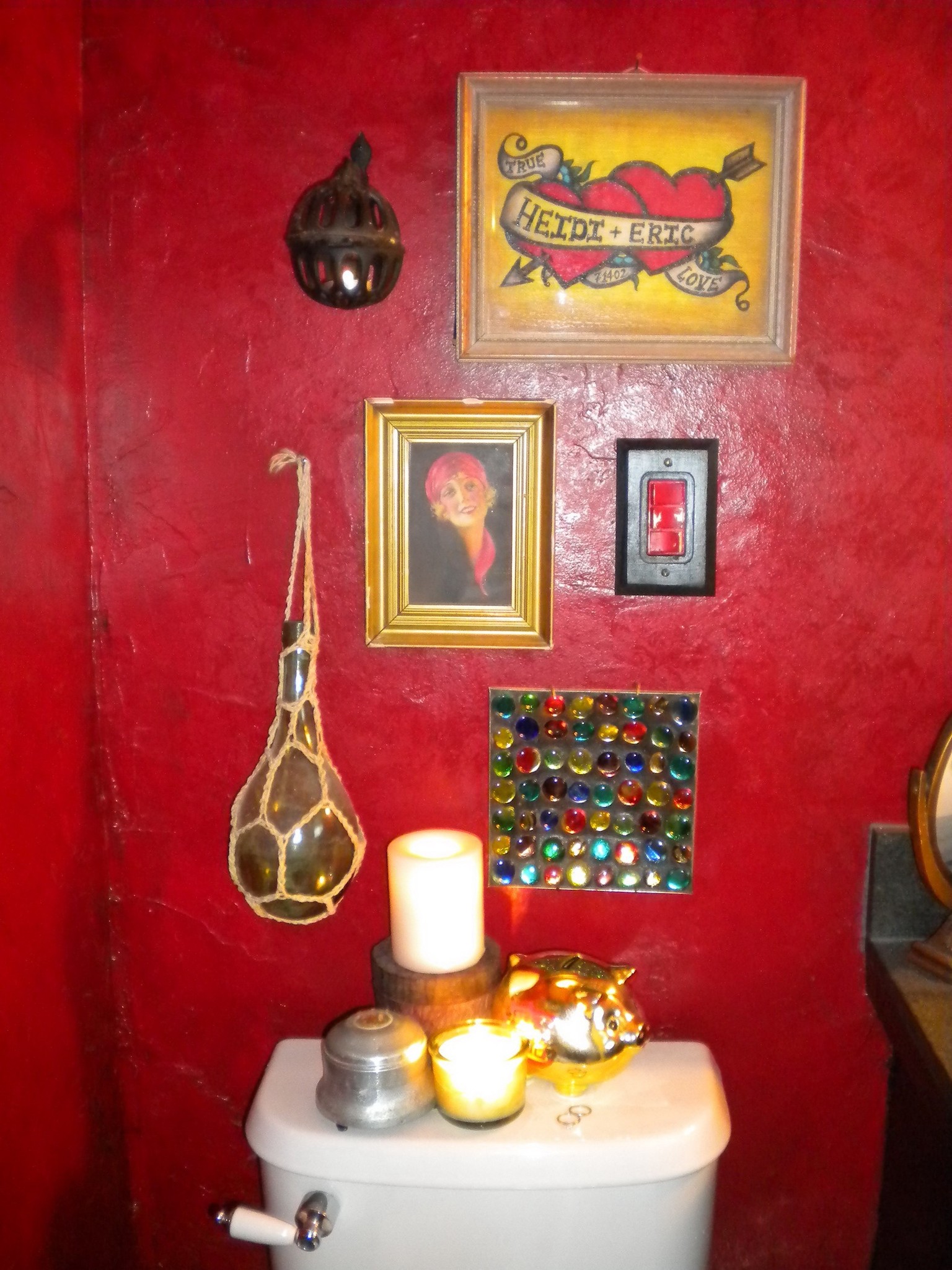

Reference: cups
[428,1018,531,1129]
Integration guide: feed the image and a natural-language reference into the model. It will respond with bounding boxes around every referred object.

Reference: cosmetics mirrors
[904,714,952,985]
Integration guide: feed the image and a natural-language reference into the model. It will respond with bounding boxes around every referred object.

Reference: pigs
[489,949,656,1100]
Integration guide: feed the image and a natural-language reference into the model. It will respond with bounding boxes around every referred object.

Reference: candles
[433,1031,526,1123]
[387,829,486,973]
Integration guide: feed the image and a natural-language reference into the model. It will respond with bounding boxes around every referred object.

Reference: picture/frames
[456,67,805,365]
[362,397,556,650]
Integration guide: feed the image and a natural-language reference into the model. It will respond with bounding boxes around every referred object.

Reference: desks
[863,941,952,1270]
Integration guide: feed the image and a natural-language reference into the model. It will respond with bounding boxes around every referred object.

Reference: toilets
[209,1034,734,1270]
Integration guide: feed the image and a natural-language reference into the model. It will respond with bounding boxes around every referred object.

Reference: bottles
[232,621,359,920]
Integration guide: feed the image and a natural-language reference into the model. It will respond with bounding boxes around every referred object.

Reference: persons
[409,451,511,606]
